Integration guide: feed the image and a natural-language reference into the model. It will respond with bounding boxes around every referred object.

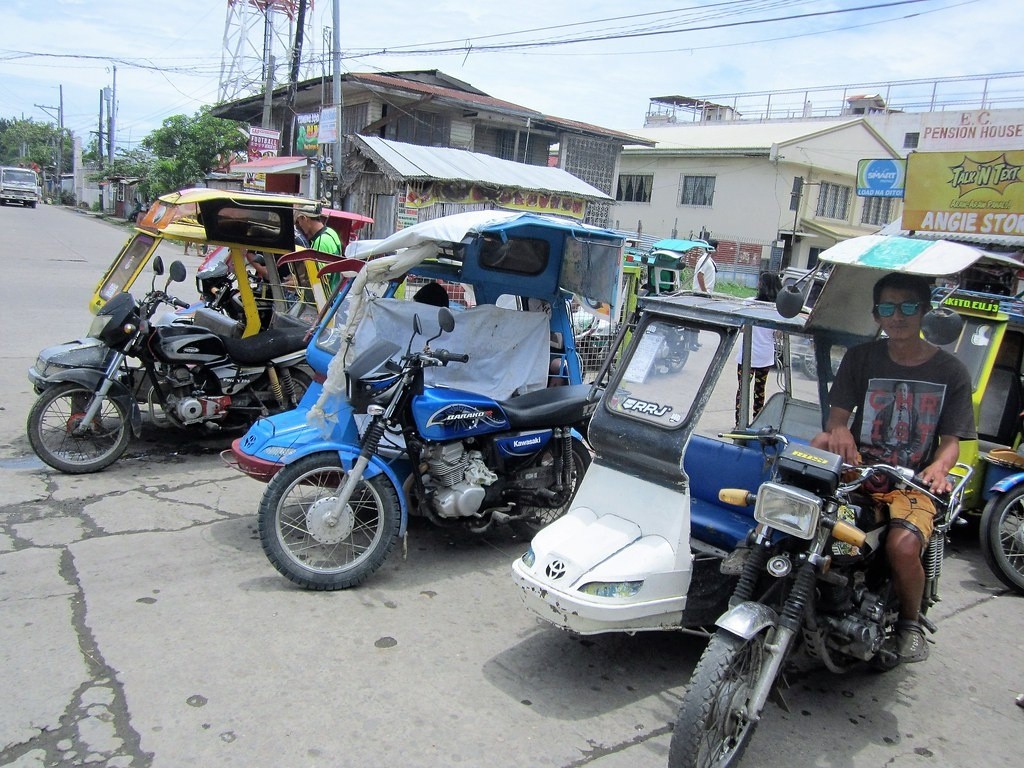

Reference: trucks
[0,168,43,209]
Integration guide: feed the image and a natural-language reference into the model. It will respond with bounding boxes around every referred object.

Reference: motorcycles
[21,187,368,478]
[511,234,1024,768]
[214,211,604,587]
[773,232,1024,599]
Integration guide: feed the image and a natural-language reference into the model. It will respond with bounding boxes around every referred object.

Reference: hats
[298,205,322,218]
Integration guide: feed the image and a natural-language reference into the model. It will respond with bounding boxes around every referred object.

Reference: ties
[898,410,902,445]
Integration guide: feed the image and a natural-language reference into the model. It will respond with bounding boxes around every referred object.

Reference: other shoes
[689,343,702,352]
[720,540,753,574]
[898,622,929,663]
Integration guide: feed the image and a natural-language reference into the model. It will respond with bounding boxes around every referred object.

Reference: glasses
[876,299,920,317]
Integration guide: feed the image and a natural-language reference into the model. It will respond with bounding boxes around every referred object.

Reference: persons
[184,241,206,257]
[37,185,41,205]
[689,240,720,352]
[129,198,143,222]
[810,272,977,663]
[735,269,783,428]
[246,250,291,291]
[281,204,342,308]
[872,382,924,466]
[529,298,568,388]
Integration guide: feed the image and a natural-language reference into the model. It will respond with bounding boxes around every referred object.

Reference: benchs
[681,434,789,555]
[742,428,813,459]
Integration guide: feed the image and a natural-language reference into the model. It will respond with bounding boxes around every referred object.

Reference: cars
[778,264,829,313]
